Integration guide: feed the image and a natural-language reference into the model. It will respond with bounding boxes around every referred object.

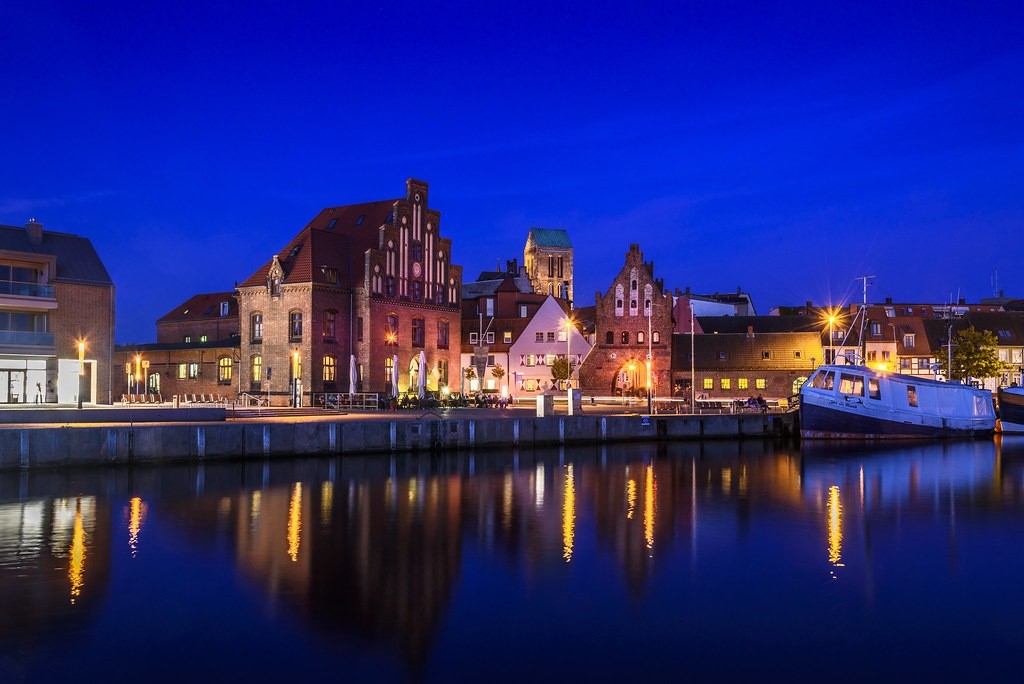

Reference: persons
[747,393,771,414]
[319,394,513,411]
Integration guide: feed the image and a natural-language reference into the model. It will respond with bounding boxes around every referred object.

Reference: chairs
[745,401,751,408]
[379,399,513,411]
[735,401,744,407]
[716,402,726,408]
[653,401,683,410]
[709,402,717,409]
[703,402,712,409]
[697,402,705,409]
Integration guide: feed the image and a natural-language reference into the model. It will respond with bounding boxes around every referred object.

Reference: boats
[996,365,1024,416]
[799,305,998,440]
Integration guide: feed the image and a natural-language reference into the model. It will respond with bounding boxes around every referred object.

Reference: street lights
[829,314,836,365]
[564,321,572,381]
